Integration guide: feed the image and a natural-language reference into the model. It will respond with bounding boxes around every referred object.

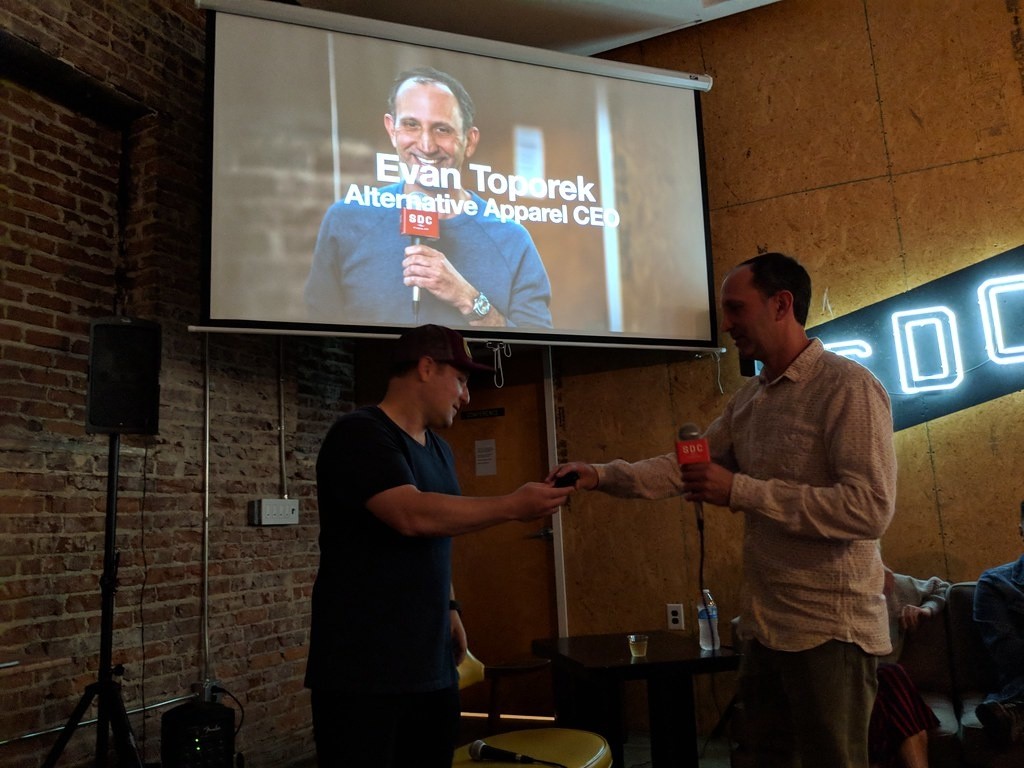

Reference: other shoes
[975,699,1024,751]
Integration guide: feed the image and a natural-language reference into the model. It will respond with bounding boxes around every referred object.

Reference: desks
[532,632,737,768]
[452,728,614,768]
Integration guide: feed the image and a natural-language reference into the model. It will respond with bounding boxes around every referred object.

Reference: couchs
[898,578,1024,768]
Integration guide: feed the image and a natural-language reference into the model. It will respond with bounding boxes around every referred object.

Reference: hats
[393,324,498,376]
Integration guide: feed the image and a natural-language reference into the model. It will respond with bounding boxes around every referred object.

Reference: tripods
[38,434,143,768]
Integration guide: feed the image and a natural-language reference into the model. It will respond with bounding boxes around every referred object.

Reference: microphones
[674,422,711,522]
[468,740,535,764]
[400,190,440,304]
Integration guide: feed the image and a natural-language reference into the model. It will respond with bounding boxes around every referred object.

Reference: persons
[304,67,555,332]
[545,249,899,768]
[973,504,1024,766]
[305,321,576,767]
[869,564,941,768]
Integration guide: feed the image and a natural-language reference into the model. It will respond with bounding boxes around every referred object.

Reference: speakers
[83,313,164,438]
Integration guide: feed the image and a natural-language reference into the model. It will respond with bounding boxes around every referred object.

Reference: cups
[628,634,649,657]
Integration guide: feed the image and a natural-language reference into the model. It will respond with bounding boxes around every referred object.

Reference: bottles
[697,589,721,652]
